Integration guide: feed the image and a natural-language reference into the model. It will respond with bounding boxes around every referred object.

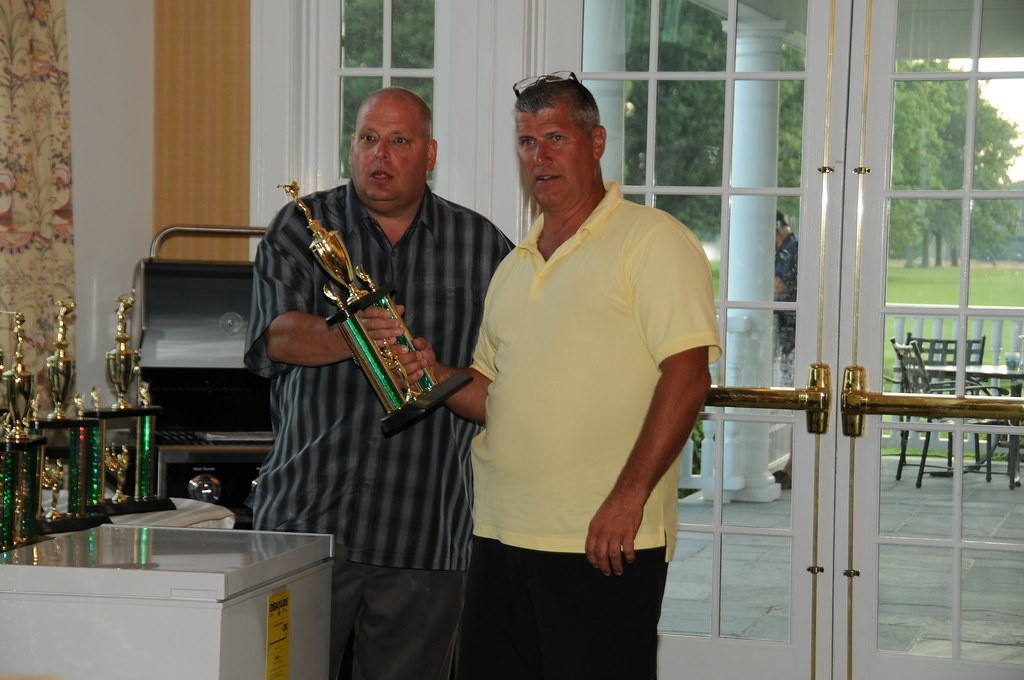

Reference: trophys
[278,181,475,436]
[0,290,175,554]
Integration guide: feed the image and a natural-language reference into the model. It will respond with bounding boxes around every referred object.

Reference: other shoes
[773,470,792,490]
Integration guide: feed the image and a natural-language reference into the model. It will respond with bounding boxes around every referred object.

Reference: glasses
[513,70,581,101]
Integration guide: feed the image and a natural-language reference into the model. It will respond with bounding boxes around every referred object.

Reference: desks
[894,365,1024,482]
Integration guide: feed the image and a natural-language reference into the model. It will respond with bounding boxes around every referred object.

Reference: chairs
[902,332,988,470]
[891,339,1018,490]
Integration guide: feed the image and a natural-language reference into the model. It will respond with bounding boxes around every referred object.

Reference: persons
[772,212,799,489]
[242,87,514,680]
[389,71,722,680]
